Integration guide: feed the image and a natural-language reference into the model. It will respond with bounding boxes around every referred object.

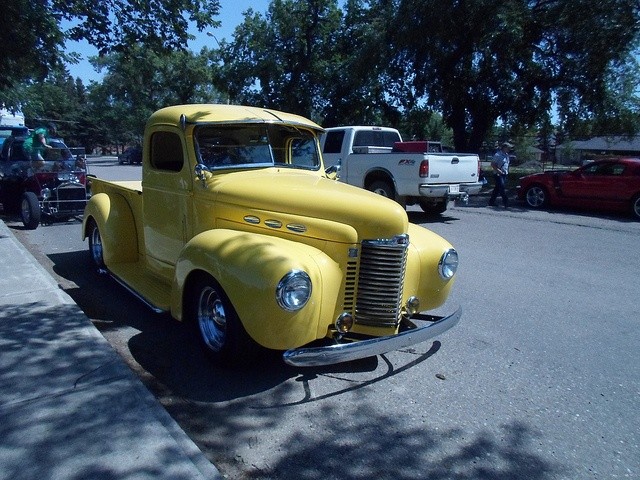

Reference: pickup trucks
[81,104,465,373]
[291,126,487,222]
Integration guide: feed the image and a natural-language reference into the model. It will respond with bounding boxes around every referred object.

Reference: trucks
[0,113,29,152]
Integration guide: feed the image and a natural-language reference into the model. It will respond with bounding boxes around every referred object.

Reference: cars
[118,144,142,166]
[516,156,639,222]
[1,137,86,228]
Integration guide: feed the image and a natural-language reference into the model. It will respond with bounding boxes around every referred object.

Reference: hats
[502,142,514,149]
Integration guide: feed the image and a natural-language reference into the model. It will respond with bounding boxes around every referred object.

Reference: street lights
[206,31,231,103]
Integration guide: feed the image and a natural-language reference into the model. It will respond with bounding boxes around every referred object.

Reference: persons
[31,122,56,172]
[490,142,513,209]
